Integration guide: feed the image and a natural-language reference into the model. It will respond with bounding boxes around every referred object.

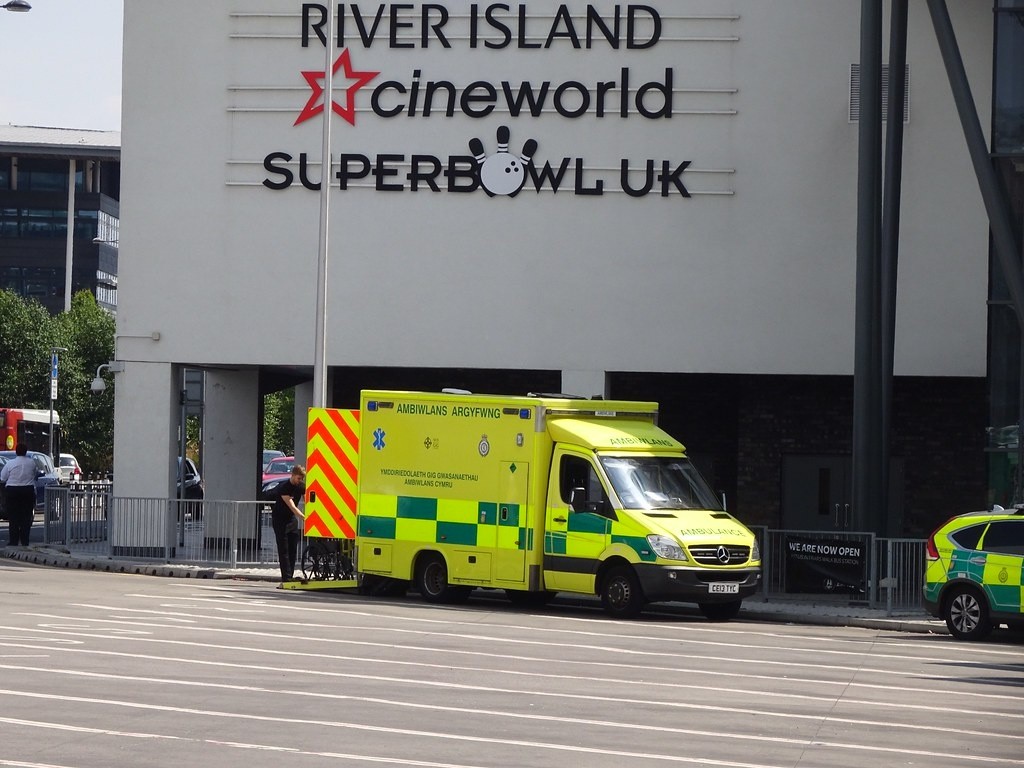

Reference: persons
[271,464,306,582]
[0,442,39,547]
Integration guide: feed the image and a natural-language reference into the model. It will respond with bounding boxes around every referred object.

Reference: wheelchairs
[301,539,356,581]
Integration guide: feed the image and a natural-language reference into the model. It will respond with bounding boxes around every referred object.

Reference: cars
[175,458,204,521]
[263,448,295,500]
[60,452,82,484]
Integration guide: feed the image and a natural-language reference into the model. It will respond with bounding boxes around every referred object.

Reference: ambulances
[280,388,764,621]
[920,504,1024,643]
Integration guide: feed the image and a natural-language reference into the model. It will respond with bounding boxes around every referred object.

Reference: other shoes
[22,543,29,546]
[7,542,17,546]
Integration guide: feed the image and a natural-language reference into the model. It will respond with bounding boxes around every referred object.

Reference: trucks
[1,408,62,521]
